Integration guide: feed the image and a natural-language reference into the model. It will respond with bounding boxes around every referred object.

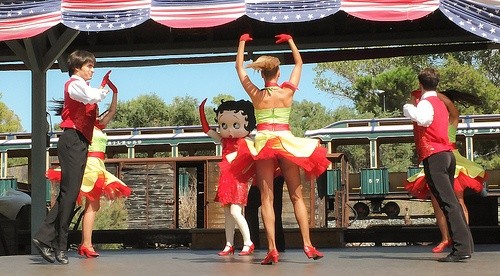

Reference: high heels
[261,250,279,265]
[77,244,99,257]
[239,243,255,256]
[432,239,452,253]
[218,245,235,256]
[303,245,324,260]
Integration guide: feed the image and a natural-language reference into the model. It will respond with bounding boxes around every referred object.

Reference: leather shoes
[53,248,69,264]
[439,251,471,262]
[32,238,55,263]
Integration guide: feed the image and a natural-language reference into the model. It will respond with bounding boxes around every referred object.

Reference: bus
[0,124,224,212]
[303,113,500,217]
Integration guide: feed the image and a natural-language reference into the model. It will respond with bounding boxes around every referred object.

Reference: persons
[45,70,132,258]
[199,97,256,256]
[235,33,330,265]
[403,68,488,262]
[32,50,111,264]
[244,165,286,252]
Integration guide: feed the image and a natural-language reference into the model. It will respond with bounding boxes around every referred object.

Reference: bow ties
[86,82,89,86]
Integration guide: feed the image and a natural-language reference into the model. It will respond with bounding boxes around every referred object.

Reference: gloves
[275,34,293,44]
[199,98,210,133]
[101,70,111,87]
[107,79,117,93]
[240,34,253,41]
